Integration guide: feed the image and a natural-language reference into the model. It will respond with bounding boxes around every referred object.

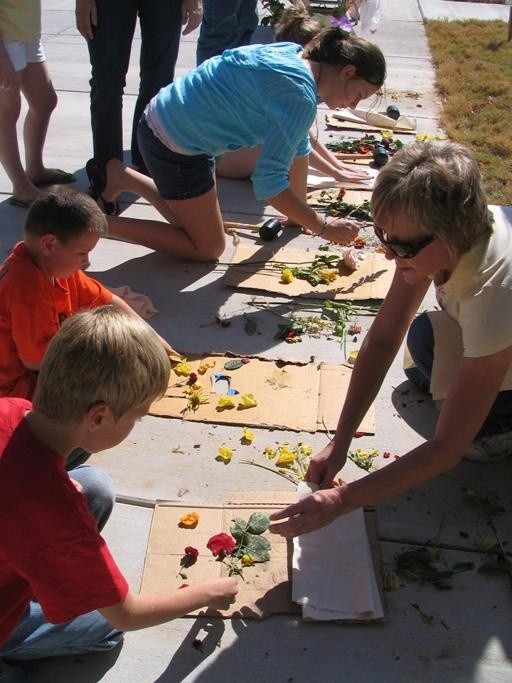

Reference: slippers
[83,157,121,216]
[41,167,76,186]
[7,193,31,210]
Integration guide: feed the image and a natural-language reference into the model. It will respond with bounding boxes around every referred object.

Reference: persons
[196,2,259,66]
[77,2,203,181]
[0,184,182,470]
[0,307,238,683]
[87,26,384,260]
[1,0,73,204]
[268,139,511,539]
[217,7,374,182]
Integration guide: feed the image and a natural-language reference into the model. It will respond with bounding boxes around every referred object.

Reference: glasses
[372,222,442,259]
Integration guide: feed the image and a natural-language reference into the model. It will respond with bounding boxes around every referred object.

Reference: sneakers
[459,429,511,462]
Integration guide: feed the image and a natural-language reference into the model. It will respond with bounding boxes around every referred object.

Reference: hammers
[377,104,399,119]
[223,219,282,237]
[333,145,388,166]
